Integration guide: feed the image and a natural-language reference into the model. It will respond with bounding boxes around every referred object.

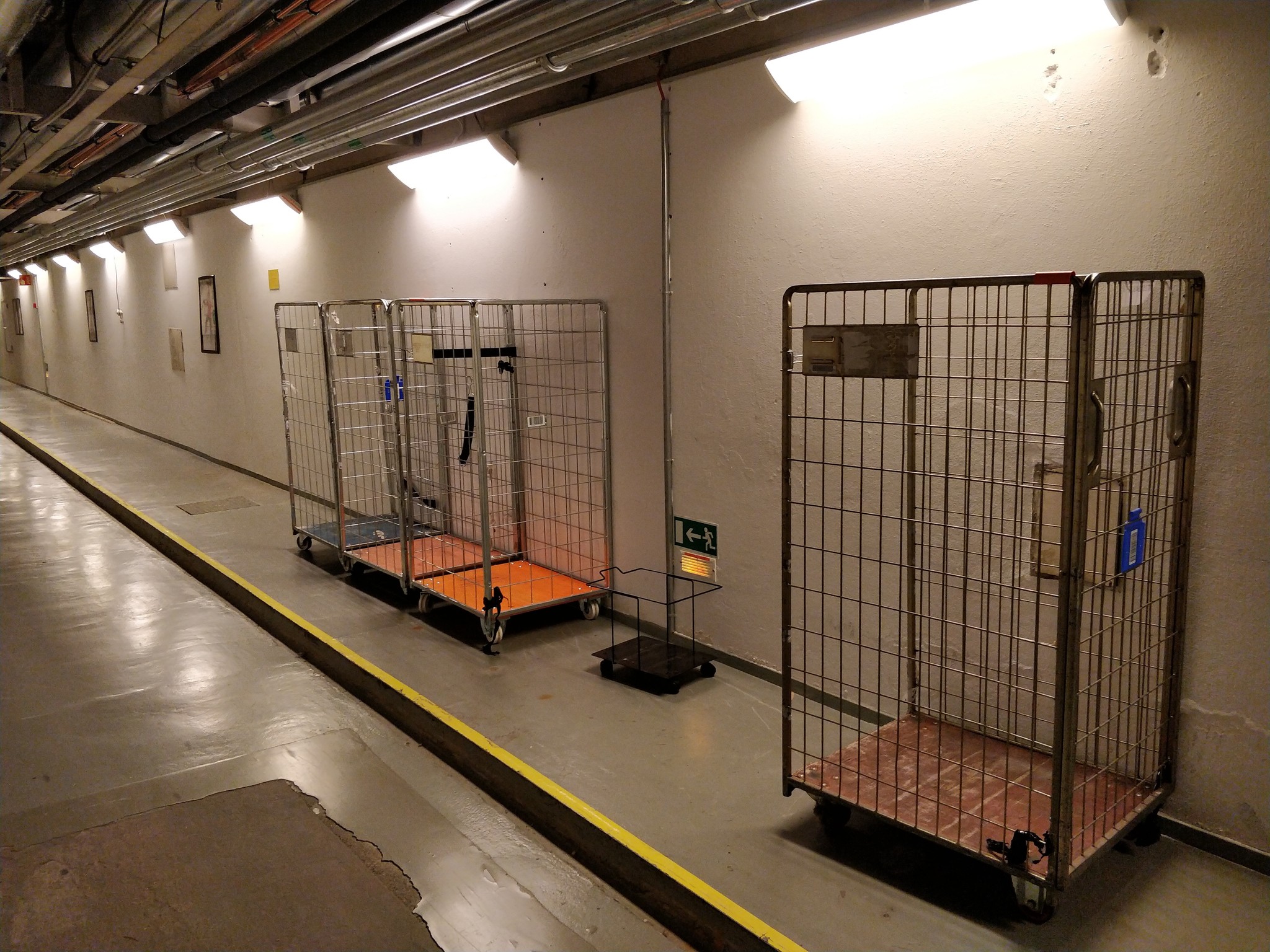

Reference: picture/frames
[12,298,24,335]
[198,275,220,354]
[85,289,98,342]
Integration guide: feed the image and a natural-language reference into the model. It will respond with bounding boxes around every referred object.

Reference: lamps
[142,217,188,245]
[24,262,48,277]
[386,132,519,190]
[51,254,82,270]
[88,240,126,261]
[229,192,303,226]
[7,268,23,280]
[763,0,1129,104]
